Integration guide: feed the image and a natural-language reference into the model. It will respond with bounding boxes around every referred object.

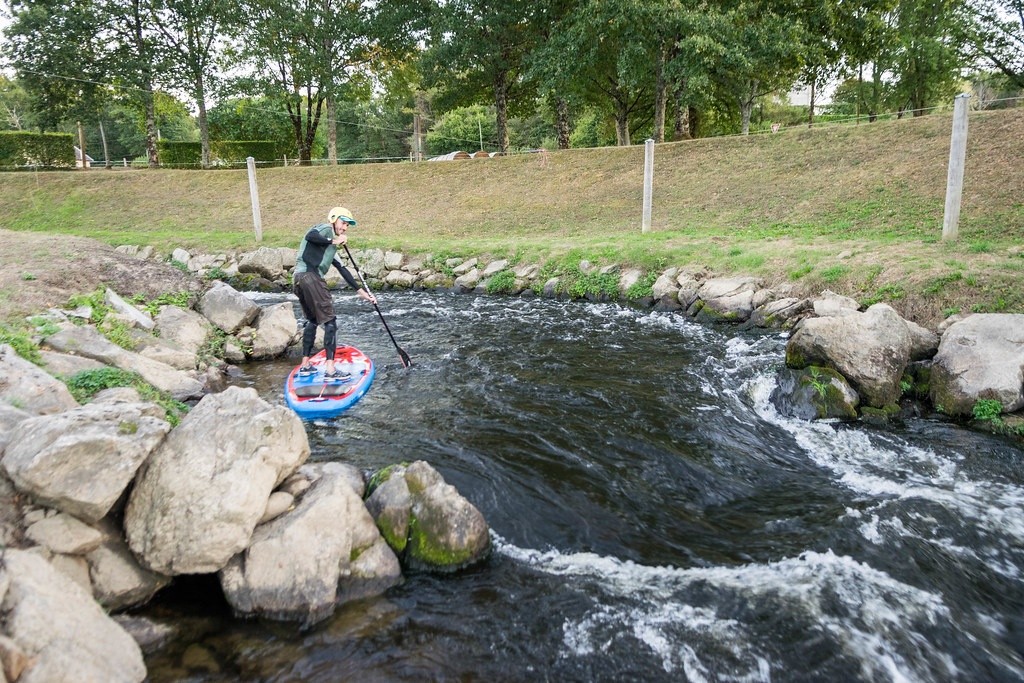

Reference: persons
[291,206,377,381]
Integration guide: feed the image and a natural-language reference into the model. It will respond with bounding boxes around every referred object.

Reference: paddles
[342,240,411,368]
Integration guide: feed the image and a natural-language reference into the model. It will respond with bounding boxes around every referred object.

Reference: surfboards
[285,342,376,419]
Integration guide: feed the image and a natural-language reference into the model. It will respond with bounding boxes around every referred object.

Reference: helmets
[328,207,356,226]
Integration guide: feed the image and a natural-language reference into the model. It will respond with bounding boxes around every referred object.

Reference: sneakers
[300,364,318,376]
[323,367,351,382]
